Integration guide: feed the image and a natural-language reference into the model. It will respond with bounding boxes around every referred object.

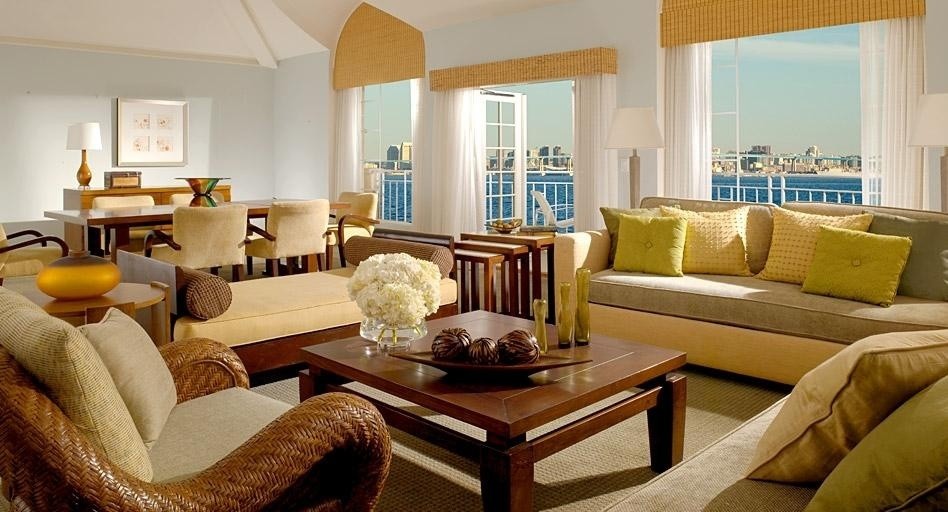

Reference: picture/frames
[117,96,191,167]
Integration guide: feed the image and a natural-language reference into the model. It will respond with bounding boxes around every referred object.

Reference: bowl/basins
[484,219,522,234]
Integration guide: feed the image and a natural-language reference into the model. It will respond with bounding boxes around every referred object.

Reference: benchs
[116,227,459,376]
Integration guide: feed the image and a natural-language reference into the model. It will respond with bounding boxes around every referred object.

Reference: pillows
[167,266,233,321]
[0,286,179,484]
[342,235,454,279]
[745,328,948,512]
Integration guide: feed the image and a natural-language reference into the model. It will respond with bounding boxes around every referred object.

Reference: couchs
[599,386,947,512]
[553,196,948,387]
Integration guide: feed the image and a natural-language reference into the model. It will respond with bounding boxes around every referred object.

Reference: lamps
[907,93,948,213]
[602,106,667,210]
[66,122,103,190]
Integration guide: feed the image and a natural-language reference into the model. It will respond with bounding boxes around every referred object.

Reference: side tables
[0,276,172,347]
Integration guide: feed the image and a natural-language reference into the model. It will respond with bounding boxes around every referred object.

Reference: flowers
[347,251,443,346]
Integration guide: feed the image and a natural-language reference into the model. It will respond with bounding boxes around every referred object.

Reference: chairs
[0,330,392,512]
[0,222,69,286]
[90,190,379,281]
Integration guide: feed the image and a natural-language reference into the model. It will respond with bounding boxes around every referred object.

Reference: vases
[34,248,123,301]
[360,313,428,352]
[532,267,591,355]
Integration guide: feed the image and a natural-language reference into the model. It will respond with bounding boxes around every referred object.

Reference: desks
[452,232,555,326]
[63,184,232,251]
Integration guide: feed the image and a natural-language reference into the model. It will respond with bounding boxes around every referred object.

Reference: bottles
[532,298,549,355]
[557,268,593,347]
